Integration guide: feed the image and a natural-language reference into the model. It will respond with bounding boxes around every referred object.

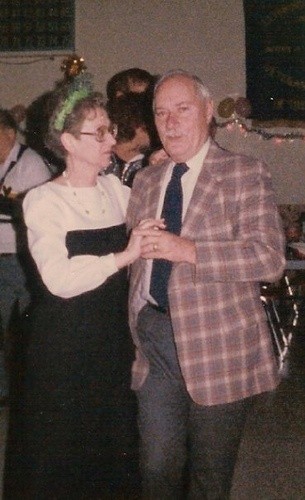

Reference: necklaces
[64,171,107,221]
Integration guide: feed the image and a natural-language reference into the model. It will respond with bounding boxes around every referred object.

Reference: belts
[147,299,169,314]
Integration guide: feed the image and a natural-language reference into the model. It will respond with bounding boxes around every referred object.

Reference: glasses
[70,123,118,142]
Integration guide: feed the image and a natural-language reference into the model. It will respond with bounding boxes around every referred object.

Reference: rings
[153,243,159,252]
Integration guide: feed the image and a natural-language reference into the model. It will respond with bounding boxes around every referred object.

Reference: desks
[260,259,305,373]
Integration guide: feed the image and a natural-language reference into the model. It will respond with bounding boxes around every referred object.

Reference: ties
[149,162,190,311]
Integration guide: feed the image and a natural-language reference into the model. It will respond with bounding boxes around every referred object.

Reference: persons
[0,68,287,500]
